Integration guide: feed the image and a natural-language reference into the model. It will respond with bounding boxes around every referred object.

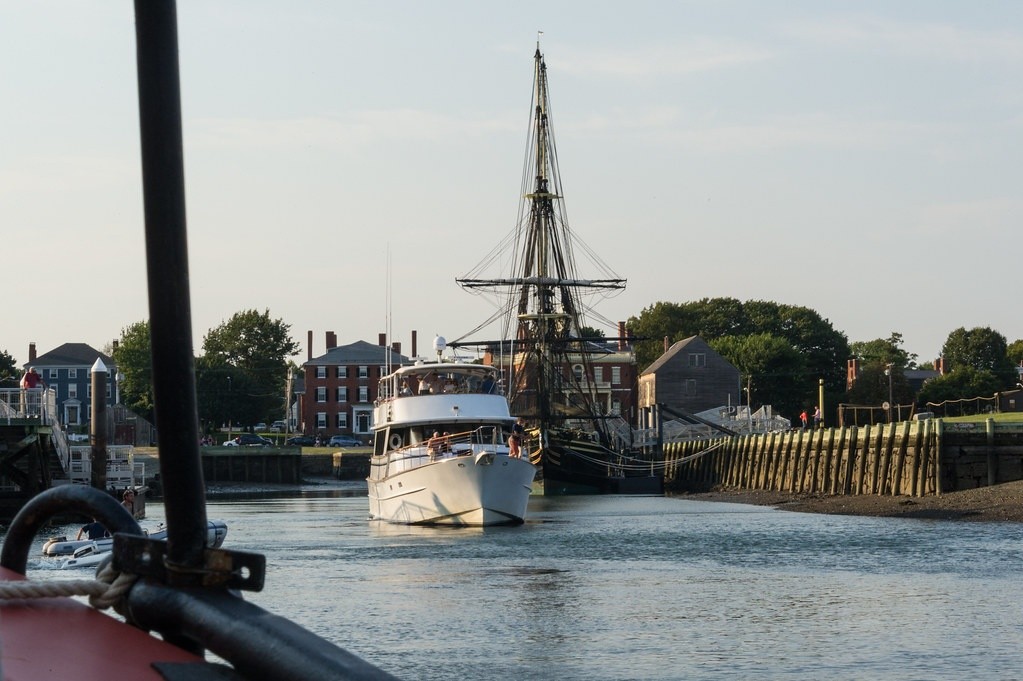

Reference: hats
[416,375,422,378]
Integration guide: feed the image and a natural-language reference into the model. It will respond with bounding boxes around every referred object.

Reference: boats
[42,536,95,556]
[365,261,537,531]
[60,520,229,568]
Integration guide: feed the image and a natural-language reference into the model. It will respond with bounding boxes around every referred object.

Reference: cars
[223,438,240,446]
[284,437,318,446]
[330,434,364,448]
[236,433,274,447]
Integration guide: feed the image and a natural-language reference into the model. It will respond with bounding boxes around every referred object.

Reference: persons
[313,433,323,447]
[426,431,441,463]
[398,372,496,396]
[439,432,453,456]
[23,366,49,420]
[508,418,526,457]
[799,409,809,431]
[199,432,215,448]
[120,489,142,522]
[811,406,822,431]
[77,515,110,541]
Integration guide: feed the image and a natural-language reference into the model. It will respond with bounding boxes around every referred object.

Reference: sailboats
[454,33,669,499]
